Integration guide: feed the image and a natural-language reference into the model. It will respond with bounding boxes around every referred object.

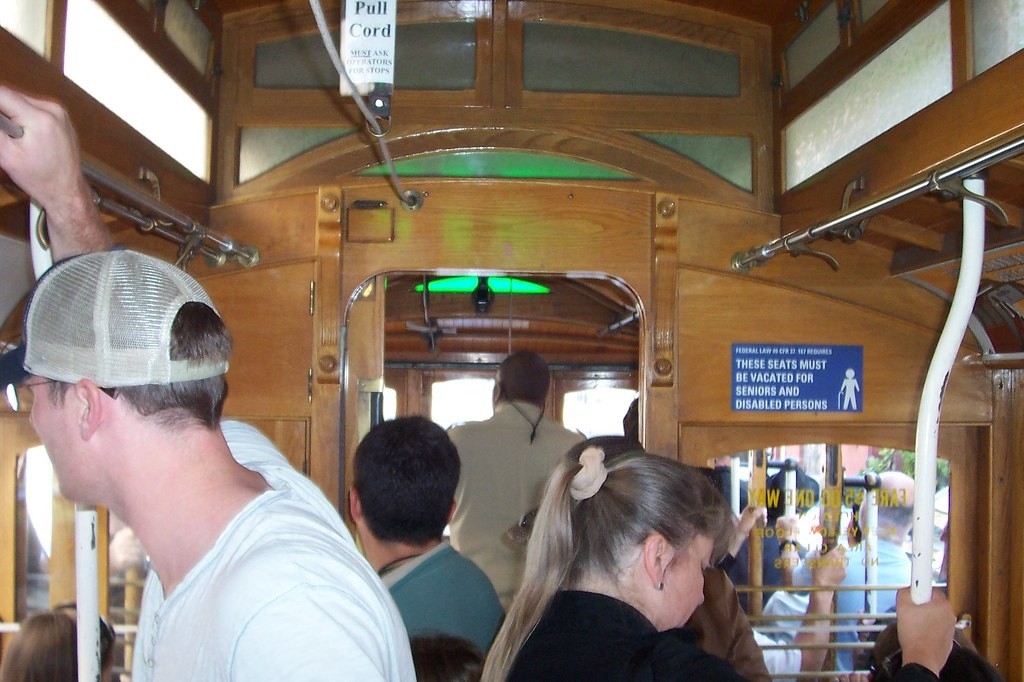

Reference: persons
[481,394,1000,681]
[411,632,486,682]
[0,79,417,682]
[349,415,505,655]
[446,349,590,617]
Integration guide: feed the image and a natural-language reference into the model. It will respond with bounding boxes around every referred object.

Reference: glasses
[51,601,115,652]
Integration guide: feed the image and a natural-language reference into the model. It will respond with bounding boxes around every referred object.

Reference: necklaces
[377,553,424,574]
[506,394,545,445]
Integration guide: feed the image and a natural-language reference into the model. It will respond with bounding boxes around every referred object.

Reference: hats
[767,468,819,513]
[0,245,230,392]
